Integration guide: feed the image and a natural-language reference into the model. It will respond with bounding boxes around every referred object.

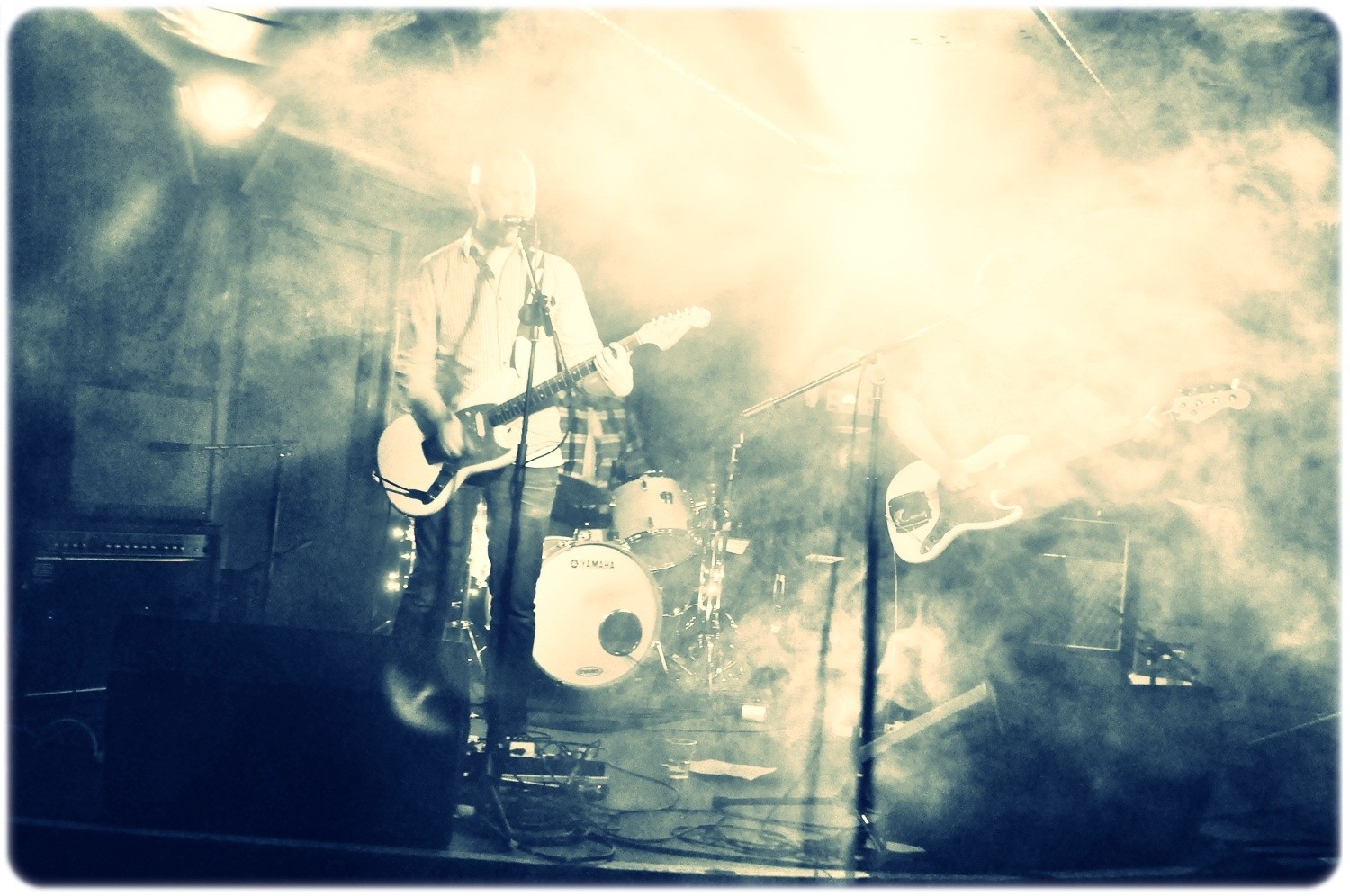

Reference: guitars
[376,302,713,519]
[883,379,1253,567]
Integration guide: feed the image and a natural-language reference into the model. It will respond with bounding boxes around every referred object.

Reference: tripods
[661,499,764,700]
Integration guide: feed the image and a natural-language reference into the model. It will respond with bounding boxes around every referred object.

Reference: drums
[609,469,698,573]
[483,534,663,691]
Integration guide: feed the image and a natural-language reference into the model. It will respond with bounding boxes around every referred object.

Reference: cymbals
[555,466,613,513]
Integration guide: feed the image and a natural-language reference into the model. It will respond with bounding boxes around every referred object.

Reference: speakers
[857,685,1230,879]
[11,528,214,698]
[1204,711,1341,859]
[57,366,221,522]
[93,614,470,852]
[1023,517,1142,672]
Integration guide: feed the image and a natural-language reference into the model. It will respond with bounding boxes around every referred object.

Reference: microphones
[147,441,204,453]
[505,214,536,227]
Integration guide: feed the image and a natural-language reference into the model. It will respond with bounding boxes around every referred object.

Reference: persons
[391,143,636,818]
